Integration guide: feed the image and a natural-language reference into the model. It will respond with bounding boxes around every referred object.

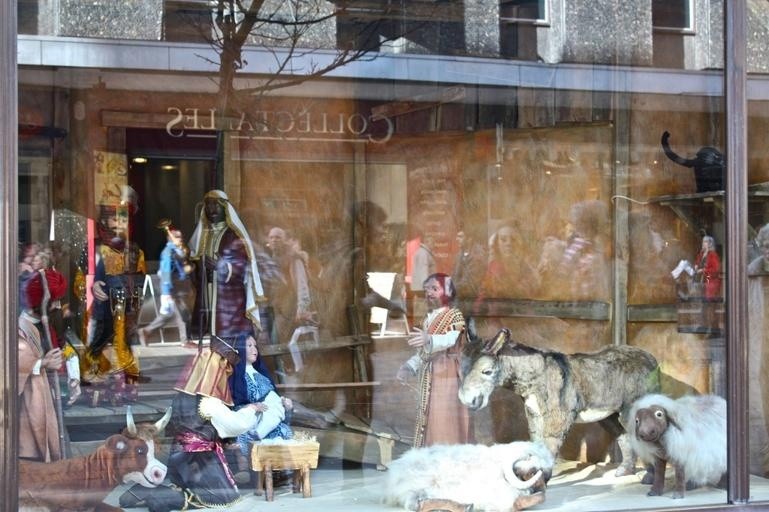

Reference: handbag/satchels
[284,322,322,376]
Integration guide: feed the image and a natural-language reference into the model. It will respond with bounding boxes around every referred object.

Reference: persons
[17,190,768,510]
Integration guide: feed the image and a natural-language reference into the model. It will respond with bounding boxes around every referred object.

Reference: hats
[569,200,609,234]
